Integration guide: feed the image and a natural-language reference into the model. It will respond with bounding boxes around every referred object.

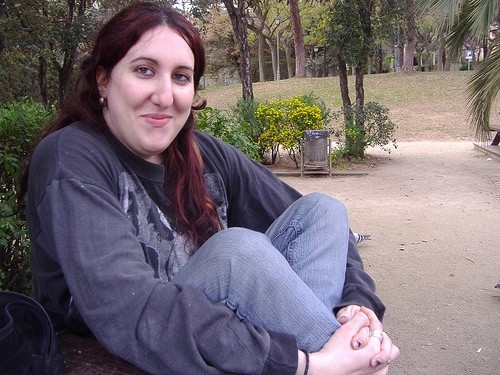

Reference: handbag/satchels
[0,289,64,375]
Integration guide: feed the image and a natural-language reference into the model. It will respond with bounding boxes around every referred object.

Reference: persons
[20,3,400,375]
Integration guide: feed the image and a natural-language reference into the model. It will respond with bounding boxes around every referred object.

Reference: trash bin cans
[302,128,329,169]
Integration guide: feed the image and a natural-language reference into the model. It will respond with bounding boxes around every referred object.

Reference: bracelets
[301,350,309,375]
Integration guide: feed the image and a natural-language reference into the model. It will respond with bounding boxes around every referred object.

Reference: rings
[369,329,382,342]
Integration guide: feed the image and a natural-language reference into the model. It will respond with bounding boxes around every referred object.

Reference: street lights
[274,13,280,80]
[314,44,319,77]
